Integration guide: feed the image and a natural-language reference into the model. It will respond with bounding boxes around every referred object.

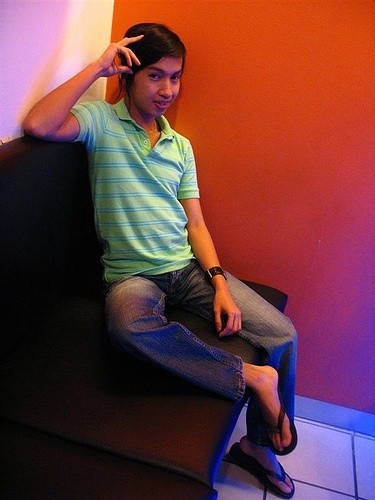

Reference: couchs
[0,131,288,500]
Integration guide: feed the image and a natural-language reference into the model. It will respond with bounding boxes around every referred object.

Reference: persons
[22,21,297,500]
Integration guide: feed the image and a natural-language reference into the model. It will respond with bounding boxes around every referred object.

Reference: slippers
[259,386,297,455]
[229,442,295,498]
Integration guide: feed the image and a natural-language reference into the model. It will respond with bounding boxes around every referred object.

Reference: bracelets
[206,265,227,285]
